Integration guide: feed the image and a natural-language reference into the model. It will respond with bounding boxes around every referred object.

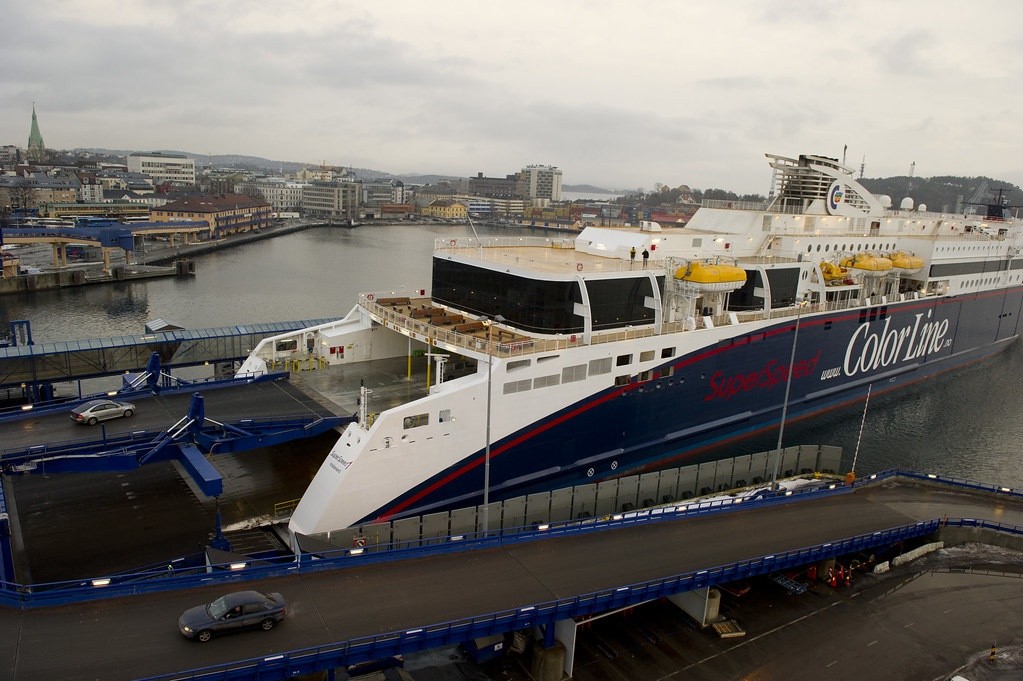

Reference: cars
[68,398,137,426]
[178,590,288,643]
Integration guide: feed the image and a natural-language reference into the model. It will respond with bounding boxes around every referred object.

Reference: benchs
[376,297,533,352]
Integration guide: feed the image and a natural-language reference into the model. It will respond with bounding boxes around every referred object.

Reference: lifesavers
[450,240,456,246]
[367,294,373,300]
[576,263,584,271]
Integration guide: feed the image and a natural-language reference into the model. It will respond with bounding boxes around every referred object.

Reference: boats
[889,250,926,276]
[673,259,747,294]
[838,250,893,277]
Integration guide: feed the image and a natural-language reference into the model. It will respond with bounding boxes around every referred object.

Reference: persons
[642,249,649,267]
[226,606,241,618]
[630,247,636,264]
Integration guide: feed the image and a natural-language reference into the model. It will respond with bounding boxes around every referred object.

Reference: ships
[223,150,1023,545]
